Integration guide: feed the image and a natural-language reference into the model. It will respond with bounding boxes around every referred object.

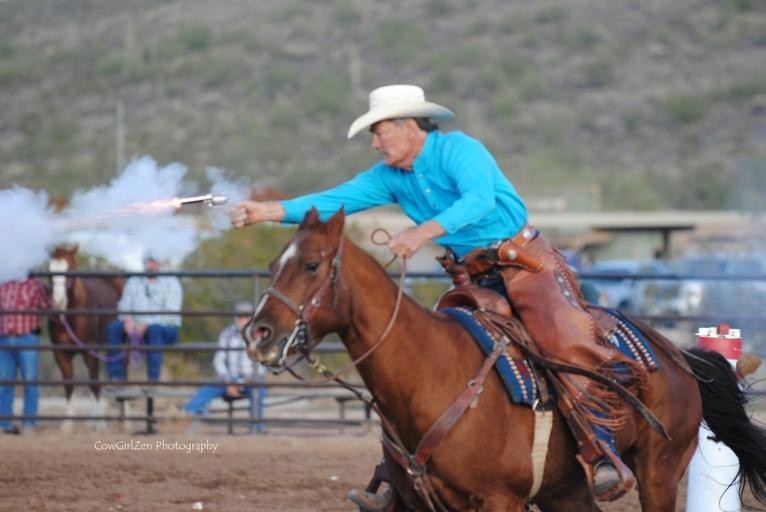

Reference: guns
[179,194,238,217]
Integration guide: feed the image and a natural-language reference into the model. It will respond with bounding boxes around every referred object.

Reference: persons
[0,270,59,437]
[218,83,631,512]
[176,300,268,439]
[101,246,184,395]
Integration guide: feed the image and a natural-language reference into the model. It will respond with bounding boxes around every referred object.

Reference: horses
[32,243,134,404]
[239,200,765,512]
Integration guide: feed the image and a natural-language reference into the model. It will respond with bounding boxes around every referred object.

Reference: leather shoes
[347,480,400,512]
[591,457,636,503]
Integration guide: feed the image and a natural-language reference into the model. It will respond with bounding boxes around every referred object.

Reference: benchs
[116,386,375,437]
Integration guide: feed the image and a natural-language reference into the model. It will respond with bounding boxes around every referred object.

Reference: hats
[234,302,252,314]
[142,245,161,263]
[346,83,455,140]
